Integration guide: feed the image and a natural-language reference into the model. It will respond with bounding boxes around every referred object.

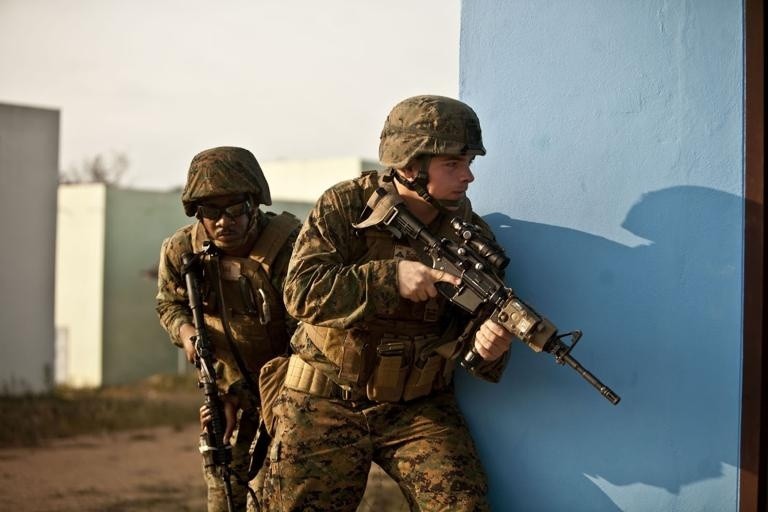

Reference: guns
[177,255,250,512]
[358,190,621,407]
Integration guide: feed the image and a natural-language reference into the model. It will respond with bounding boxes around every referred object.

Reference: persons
[246,91,515,512]
[154,143,303,511]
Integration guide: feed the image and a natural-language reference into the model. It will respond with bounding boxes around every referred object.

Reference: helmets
[378,94,487,170]
[181,146,272,217]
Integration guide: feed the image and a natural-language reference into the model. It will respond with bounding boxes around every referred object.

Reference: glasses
[195,200,251,220]
